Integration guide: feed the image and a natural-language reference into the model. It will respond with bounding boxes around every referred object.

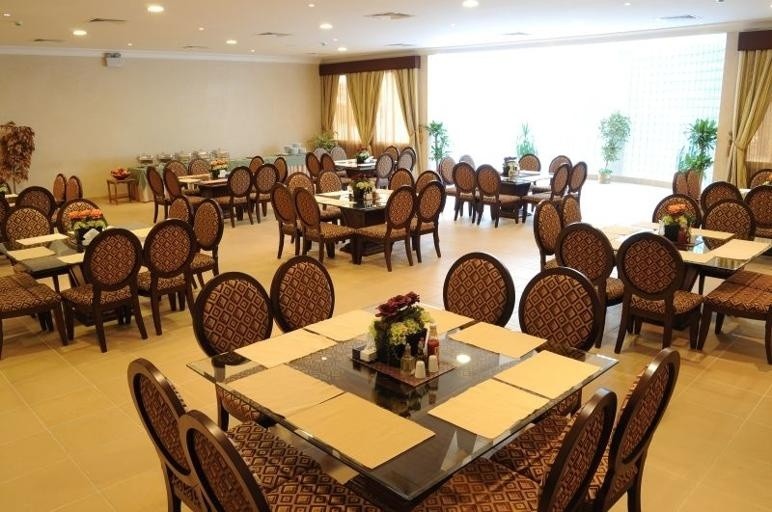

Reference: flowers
[208,159,229,171]
[502,157,517,176]
[368,293,434,346]
[662,204,698,243]
[68,207,108,237]
[353,149,369,159]
[352,175,375,195]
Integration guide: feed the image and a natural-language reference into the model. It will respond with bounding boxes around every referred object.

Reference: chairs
[476,164,521,228]
[51,175,83,227]
[383,146,397,161]
[165,159,189,189]
[367,154,393,189]
[533,200,562,268]
[521,163,570,223]
[147,195,194,312]
[355,185,418,272]
[125,217,195,335]
[215,166,254,227]
[16,186,56,218]
[56,198,103,235]
[344,386,616,509]
[189,158,209,189]
[320,152,352,190]
[672,172,690,196]
[743,184,772,238]
[518,267,601,426]
[650,194,702,230]
[52,172,66,205]
[1,273,47,330]
[274,156,288,183]
[613,231,705,353]
[330,146,347,177]
[748,168,772,191]
[388,168,414,190]
[174,410,382,512]
[271,184,310,261]
[401,145,416,166]
[699,181,745,212]
[544,160,587,201]
[193,272,276,432]
[286,171,345,248]
[189,198,224,290]
[685,169,700,199]
[724,265,772,292]
[453,162,480,224]
[553,221,634,349]
[146,166,171,223]
[126,358,321,511]
[393,150,415,174]
[240,164,279,224]
[491,348,681,510]
[3,205,54,267]
[415,170,443,195]
[244,155,264,212]
[517,154,541,185]
[532,155,573,212]
[558,193,581,228]
[0,196,10,225]
[305,152,319,181]
[314,147,330,164]
[0,282,68,360]
[438,155,463,216]
[699,198,755,296]
[316,169,345,244]
[458,154,475,170]
[271,256,335,334]
[442,252,515,327]
[58,228,147,352]
[162,167,203,205]
[294,186,357,264]
[696,277,772,368]
[409,180,446,263]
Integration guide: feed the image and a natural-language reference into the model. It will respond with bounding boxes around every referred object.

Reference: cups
[415,362,425,377]
[428,355,439,372]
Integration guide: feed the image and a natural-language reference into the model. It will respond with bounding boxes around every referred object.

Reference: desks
[333,159,398,178]
[186,298,621,503]
[312,189,396,227]
[177,170,232,197]
[500,169,554,186]
[106,179,135,204]
[604,220,771,296]
[0,225,153,293]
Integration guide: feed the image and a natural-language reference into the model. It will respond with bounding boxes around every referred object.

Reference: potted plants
[597,112,632,183]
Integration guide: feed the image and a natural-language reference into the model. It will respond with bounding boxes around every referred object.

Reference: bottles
[417,341,424,363]
[400,343,415,376]
[429,378,439,404]
[427,325,439,367]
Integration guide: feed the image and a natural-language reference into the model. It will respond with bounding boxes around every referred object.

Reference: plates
[284,144,306,153]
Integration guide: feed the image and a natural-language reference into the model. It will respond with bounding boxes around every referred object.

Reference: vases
[212,168,227,180]
[357,157,364,163]
[664,224,681,240]
[376,333,421,365]
[353,191,373,208]
[78,227,102,252]
[503,166,517,177]
